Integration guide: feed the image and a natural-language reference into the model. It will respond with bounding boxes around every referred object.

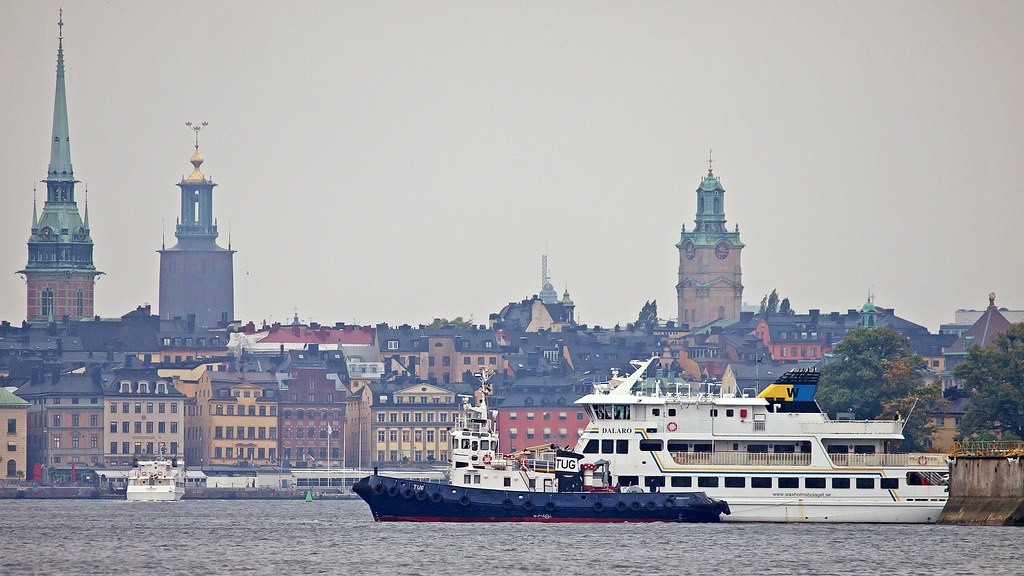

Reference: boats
[448,350,949,524]
[126,447,185,501]
[353,368,730,523]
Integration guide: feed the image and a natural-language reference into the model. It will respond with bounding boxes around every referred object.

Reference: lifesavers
[403,488,414,499]
[593,501,604,512]
[663,498,675,509]
[374,482,388,495]
[483,454,492,464]
[431,493,443,504]
[388,486,399,497]
[502,499,513,510]
[545,501,555,511]
[646,500,657,512]
[417,490,428,501]
[616,500,626,511]
[630,501,640,511]
[918,455,927,465]
[667,421,678,432]
[459,495,471,507]
[523,499,534,510]
